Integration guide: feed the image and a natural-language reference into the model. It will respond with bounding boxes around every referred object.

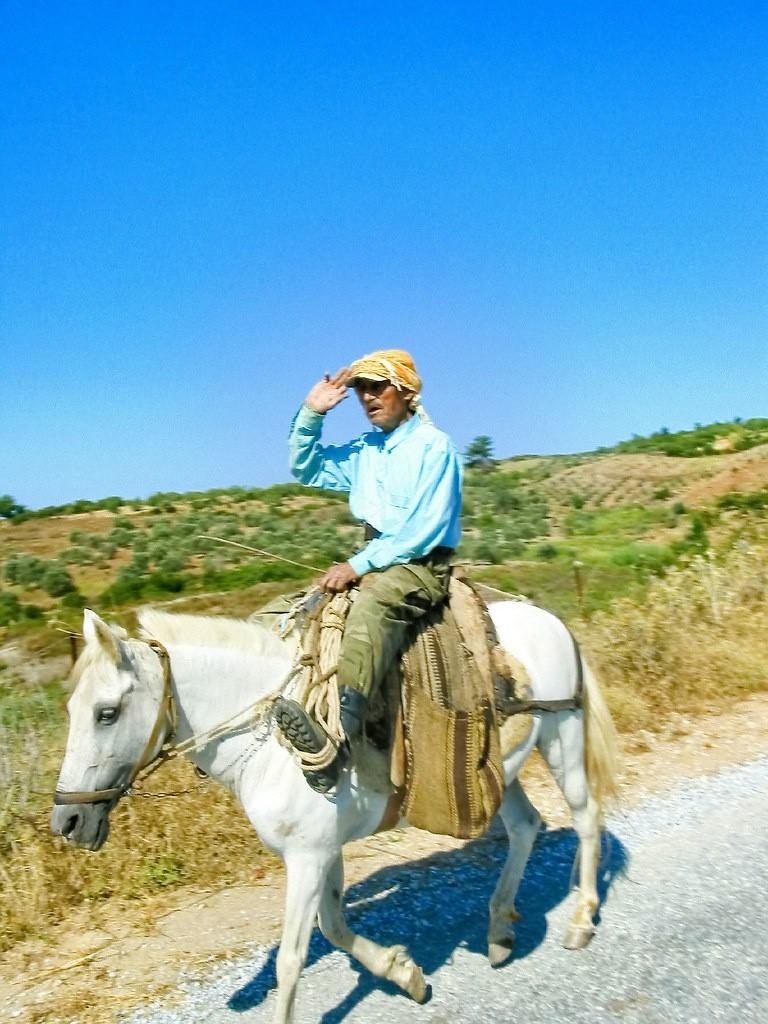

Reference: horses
[48,576,622,1024]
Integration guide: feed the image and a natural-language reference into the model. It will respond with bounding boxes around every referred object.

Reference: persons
[277,350,463,793]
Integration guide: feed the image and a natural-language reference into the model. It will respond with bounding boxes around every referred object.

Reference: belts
[366,523,453,561]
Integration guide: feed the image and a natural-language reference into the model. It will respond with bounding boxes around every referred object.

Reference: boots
[276,683,373,793]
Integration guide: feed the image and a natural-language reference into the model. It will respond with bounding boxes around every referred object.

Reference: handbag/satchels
[397,603,505,840]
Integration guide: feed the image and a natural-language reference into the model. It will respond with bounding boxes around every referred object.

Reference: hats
[346,350,423,396]
